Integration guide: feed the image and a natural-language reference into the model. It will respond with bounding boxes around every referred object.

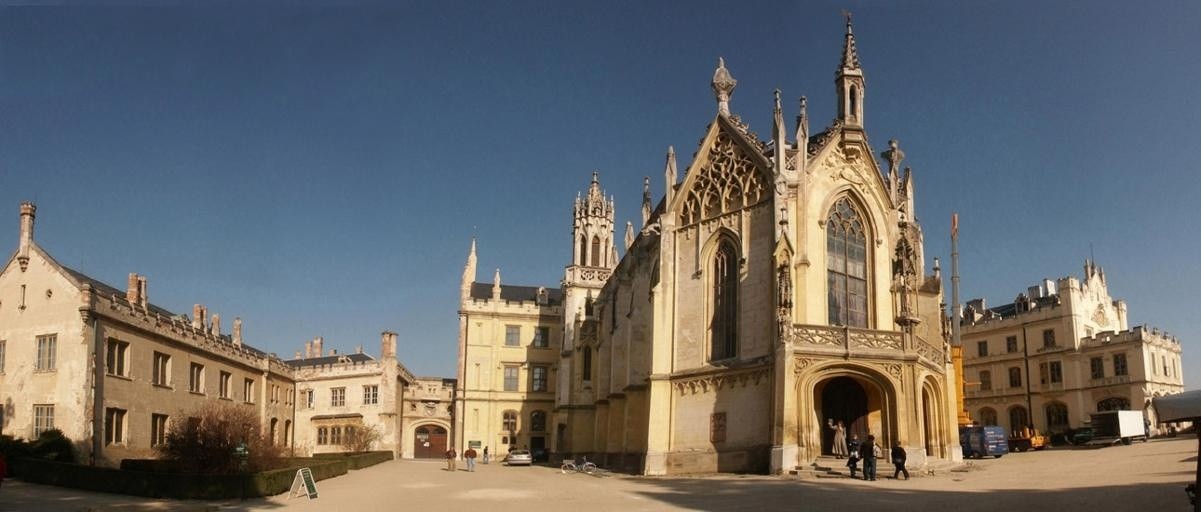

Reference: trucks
[1088,410,1152,449]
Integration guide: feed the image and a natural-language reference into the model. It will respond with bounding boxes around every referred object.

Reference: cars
[507,449,534,467]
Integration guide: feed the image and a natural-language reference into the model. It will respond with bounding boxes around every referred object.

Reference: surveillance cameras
[1152,389,1201,423]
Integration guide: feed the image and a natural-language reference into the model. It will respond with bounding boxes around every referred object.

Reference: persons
[446,445,457,471]
[891,439,911,480]
[828,418,849,460]
[483,445,491,463]
[849,433,861,457]
[464,445,478,471]
[847,449,863,478]
[860,434,881,481]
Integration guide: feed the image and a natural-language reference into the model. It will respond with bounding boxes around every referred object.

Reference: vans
[959,425,1009,460]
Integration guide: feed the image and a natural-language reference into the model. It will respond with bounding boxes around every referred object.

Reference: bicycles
[559,456,600,476]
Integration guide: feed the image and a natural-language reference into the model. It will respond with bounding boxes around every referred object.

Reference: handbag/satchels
[872,441,884,459]
